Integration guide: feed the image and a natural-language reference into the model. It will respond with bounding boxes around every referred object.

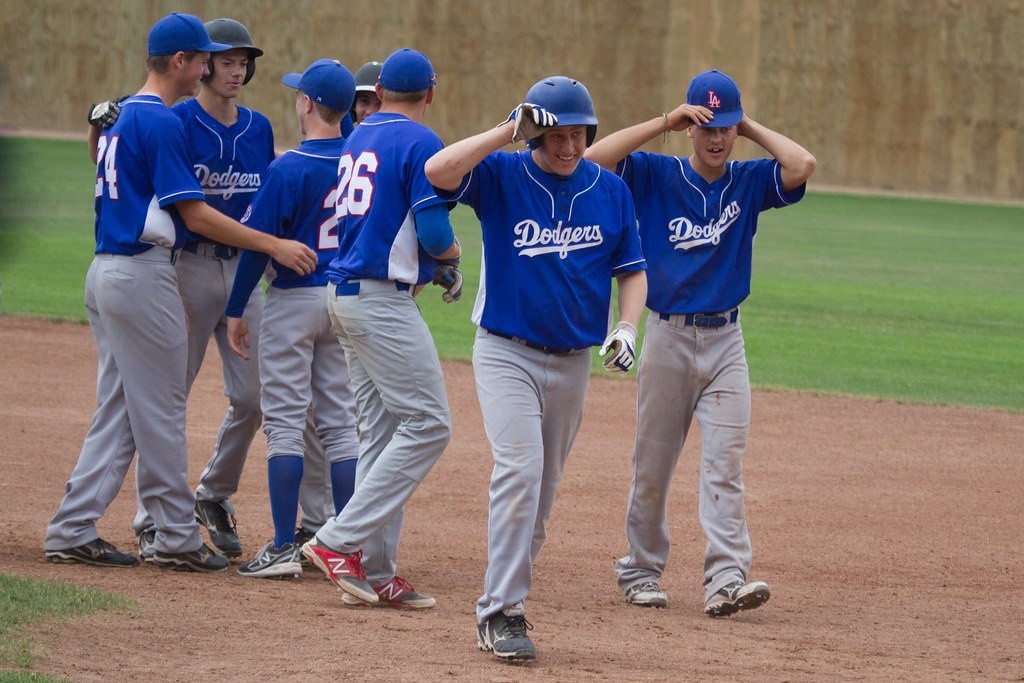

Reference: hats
[379,47,437,92]
[282,58,356,113]
[148,12,232,51]
[689,69,743,127]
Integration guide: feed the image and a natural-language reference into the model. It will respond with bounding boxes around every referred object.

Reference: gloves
[498,104,558,143]
[599,322,639,374]
[88,94,130,130]
[433,263,463,304]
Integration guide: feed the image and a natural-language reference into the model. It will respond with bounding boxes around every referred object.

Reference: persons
[88,17,275,562]
[298,48,464,608]
[425,76,648,661]
[578,70,817,618]
[224,57,356,578]
[44,11,318,573]
[349,60,383,127]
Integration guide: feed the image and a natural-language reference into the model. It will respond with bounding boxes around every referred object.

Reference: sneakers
[341,576,436,610]
[153,543,228,571]
[45,537,139,566]
[292,527,318,567]
[238,541,302,578]
[475,611,537,660]
[195,498,242,559]
[626,581,668,607]
[138,528,156,561]
[299,536,380,604]
[702,582,770,617]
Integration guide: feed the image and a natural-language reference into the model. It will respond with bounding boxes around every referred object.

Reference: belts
[489,329,566,355]
[183,243,238,260]
[335,281,411,296]
[659,309,738,329]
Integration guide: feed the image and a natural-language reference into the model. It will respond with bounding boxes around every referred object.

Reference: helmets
[523,76,597,150]
[201,18,263,83]
[355,62,384,91]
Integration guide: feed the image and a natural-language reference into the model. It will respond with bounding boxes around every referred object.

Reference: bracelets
[661,112,671,144]
